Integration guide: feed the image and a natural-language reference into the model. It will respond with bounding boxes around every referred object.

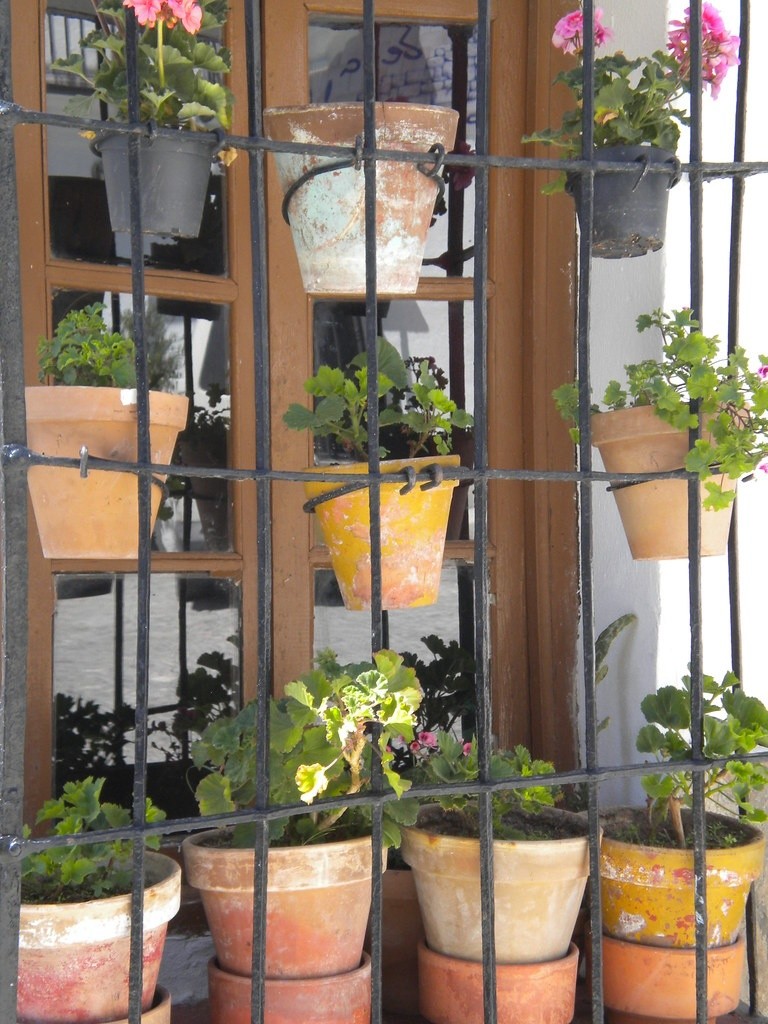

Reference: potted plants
[17,778,184,1021]
[589,667,768,950]
[283,344,471,603]
[17,300,191,560]
[186,650,411,976]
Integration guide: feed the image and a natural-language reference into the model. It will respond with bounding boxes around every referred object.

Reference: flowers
[399,730,565,828]
[72,1,240,134]
[543,0,742,154]
[552,309,767,512]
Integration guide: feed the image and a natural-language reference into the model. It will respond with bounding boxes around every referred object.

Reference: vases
[416,934,579,1024]
[206,953,372,1024]
[398,807,608,957]
[92,131,223,239]
[562,149,681,257]
[584,930,745,1024]
[579,400,750,562]
[262,103,461,299]
